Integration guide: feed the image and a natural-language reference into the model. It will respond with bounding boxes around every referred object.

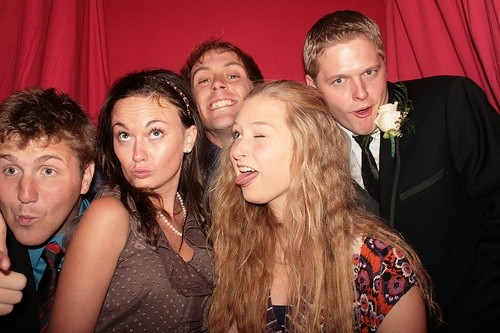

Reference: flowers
[375,102,410,158]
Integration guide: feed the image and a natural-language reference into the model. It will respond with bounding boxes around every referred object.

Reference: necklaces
[158,192,187,236]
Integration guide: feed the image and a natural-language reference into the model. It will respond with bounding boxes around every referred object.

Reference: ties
[34,240,65,333]
[353,134,380,204]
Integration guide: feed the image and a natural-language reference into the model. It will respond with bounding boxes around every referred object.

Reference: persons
[303,9,500,333]
[204,79,431,333]
[0,87,99,333]
[52,69,214,333]
[180,39,265,150]
